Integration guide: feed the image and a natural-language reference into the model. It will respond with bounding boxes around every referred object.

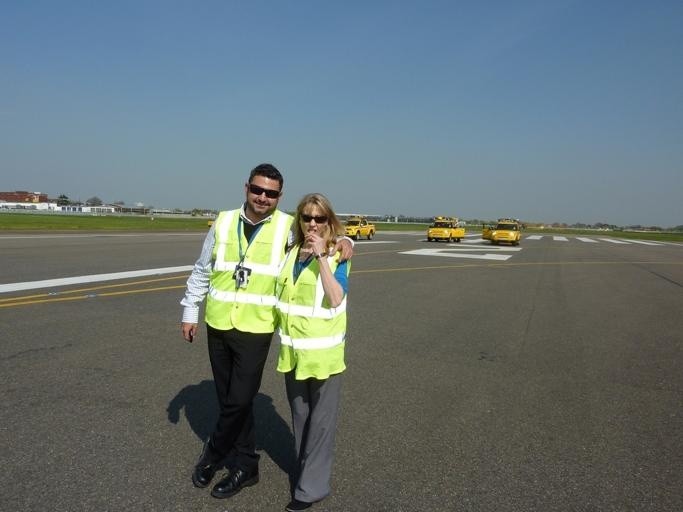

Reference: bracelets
[315,251,327,260]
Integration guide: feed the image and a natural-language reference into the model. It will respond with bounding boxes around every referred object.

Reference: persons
[275,191,353,512]
[179,163,355,500]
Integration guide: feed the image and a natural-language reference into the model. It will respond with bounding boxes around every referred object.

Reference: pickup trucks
[340,216,375,241]
[427,215,465,243]
[482,218,521,247]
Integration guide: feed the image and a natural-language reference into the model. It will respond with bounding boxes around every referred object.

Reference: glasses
[247,184,283,200]
[299,212,328,224]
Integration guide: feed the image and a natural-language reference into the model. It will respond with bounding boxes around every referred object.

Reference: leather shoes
[191,434,220,488]
[209,465,261,499]
[285,497,314,512]
